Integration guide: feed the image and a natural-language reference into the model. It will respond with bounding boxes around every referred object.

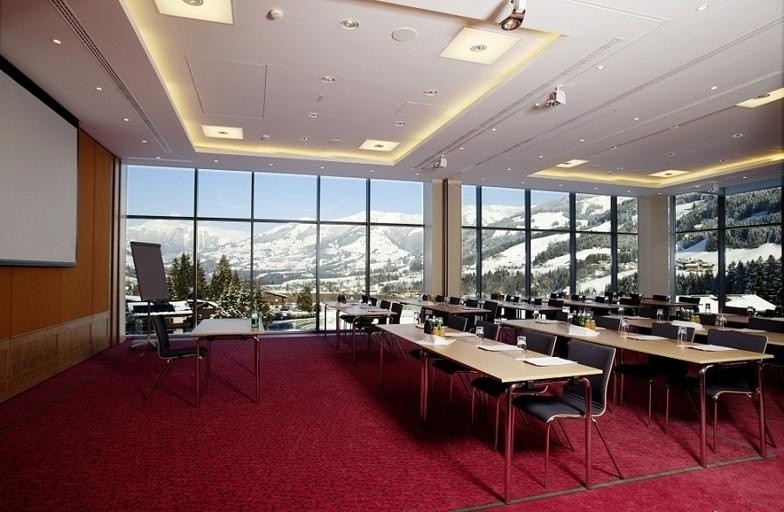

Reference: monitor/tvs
[130,241,170,302]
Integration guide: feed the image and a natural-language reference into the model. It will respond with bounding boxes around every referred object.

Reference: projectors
[545,90,566,108]
[434,154,447,169]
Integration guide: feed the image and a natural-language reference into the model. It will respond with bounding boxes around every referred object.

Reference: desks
[188,317,263,410]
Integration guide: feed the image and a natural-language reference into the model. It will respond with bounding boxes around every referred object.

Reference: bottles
[705,303,711,315]
[250,304,260,330]
[571,309,596,333]
[715,312,728,330]
[674,307,700,328]
[747,306,754,318]
[424,315,446,339]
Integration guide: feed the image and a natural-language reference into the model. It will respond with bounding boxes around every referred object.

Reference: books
[345,299,618,313]
[535,319,566,324]
[678,345,739,351]
[443,331,477,338]
[478,344,520,351]
[517,356,577,367]
[718,327,767,333]
[622,314,652,320]
[625,334,670,341]
[415,324,449,329]
[671,319,706,331]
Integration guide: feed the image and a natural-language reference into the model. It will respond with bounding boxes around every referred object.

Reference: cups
[516,336,527,354]
[618,319,629,338]
[476,327,484,342]
[347,290,672,321]
[677,326,687,346]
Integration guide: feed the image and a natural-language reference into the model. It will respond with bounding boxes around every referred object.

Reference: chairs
[142,315,207,402]
